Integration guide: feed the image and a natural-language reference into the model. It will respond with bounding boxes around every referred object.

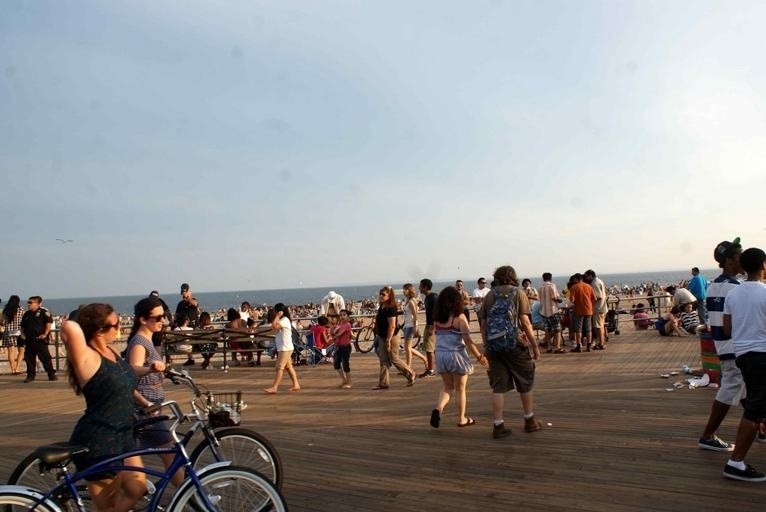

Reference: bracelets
[476,353,484,361]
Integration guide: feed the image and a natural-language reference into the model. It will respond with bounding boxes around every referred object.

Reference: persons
[471,278,489,334]
[455,279,470,323]
[122,294,224,512]
[399,281,427,376]
[686,267,708,329]
[721,247,766,485]
[226,291,379,368]
[521,269,701,354]
[475,263,544,439]
[20,296,58,384]
[427,285,487,429]
[369,287,417,390]
[57,303,165,511]
[329,309,356,391]
[694,235,765,454]
[0,294,28,376]
[417,277,439,379]
[263,301,301,395]
[150,283,225,369]
[397,297,425,315]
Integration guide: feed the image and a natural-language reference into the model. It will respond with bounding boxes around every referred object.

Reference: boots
[494,422,512,438]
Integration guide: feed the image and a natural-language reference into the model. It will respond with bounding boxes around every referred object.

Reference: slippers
[524,415,542,432]
[430,408,439,428]
[457,418,475,426]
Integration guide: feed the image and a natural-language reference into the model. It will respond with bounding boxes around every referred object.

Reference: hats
[714,237,740,262]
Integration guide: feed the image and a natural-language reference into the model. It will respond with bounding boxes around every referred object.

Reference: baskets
[207,392,242,428]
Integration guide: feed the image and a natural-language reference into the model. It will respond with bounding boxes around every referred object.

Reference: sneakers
[183,360,195,365]
[407,372,417,386]
[207,495,221,505]
[201,361,207,368]
[698,436,735,452]
[48,375,57,381]
[246,360,254,365]
[419,370,437,378]
[757,432,765,443]
[372,385,390,390]
[206,363,212,369]
[539,337,609,353]
[723,461,766,482]
[23,377,35,382]
[683,365,691,373]
[227,360,239,366]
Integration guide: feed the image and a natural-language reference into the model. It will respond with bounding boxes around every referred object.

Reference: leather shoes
[15,372,23,374]
[12,373,17,375]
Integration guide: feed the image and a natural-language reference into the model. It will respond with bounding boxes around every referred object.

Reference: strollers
[603,294,621,336]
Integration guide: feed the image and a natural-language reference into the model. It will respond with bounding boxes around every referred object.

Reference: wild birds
[56,238,74,245]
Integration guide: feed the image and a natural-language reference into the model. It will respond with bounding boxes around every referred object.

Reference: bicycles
[0,398,288,512]
[355,311,420,354]
[6,365,284,512]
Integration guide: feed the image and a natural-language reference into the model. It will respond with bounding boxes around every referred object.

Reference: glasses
[150,313,166,322]
[28,301,38,304]
[103,322,119,330]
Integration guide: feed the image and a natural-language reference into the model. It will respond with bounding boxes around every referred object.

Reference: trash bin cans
[306,332,323,365]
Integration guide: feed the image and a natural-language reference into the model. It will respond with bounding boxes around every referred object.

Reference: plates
[660,374,670,378]
[670,371,679,376]
